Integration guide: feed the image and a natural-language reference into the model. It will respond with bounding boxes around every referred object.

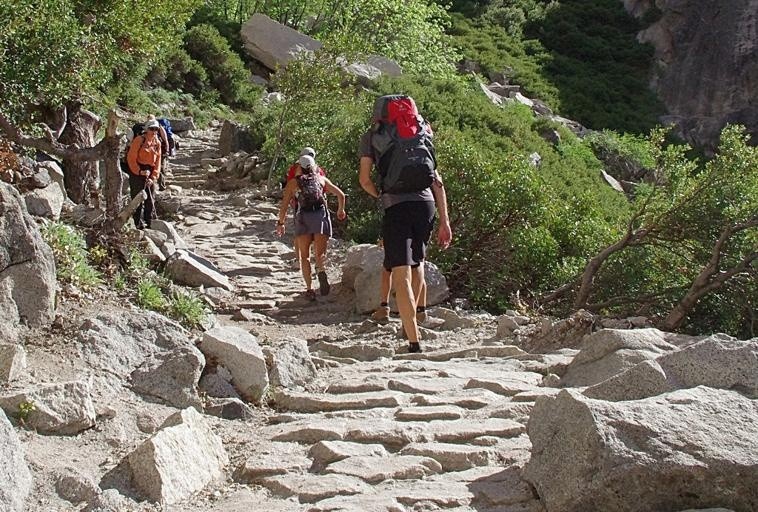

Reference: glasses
[148,127,159,131]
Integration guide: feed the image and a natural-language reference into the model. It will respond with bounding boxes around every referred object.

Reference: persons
[126,113,171,230]
[276,146,348,304]
[358,93,453,352]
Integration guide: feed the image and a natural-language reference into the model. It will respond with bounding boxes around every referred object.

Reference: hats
[299,147,316,160]
[295,155,315,170]
[145,120,160,132]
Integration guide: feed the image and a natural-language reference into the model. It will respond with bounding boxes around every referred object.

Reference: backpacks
[132,122,146,149]
[366,94,439,194]
[294,174,328,212]
[158,118,175,150]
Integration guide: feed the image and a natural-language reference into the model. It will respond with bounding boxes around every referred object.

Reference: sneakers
[415,311,427,324]
[398,344,422,353]
[371,307,390,325]
[305,291,316,301]
[316,268,330,295]
[137,220,151,230]
